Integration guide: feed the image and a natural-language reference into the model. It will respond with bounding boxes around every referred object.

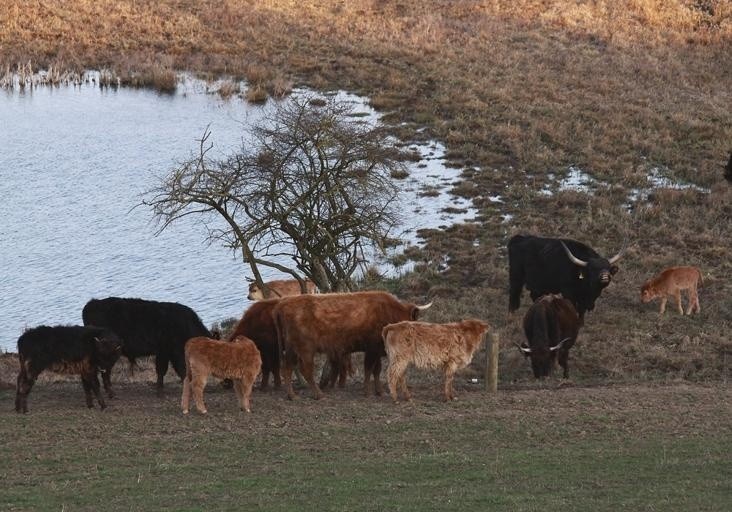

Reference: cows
[381,319,491,405]
[507,234,627,327]
[640,266,705,316]
[14,325,124,414]
[221,278,435,402]
[81,297,221,400]
[512,293,583,381]
[179,335,263,415]
